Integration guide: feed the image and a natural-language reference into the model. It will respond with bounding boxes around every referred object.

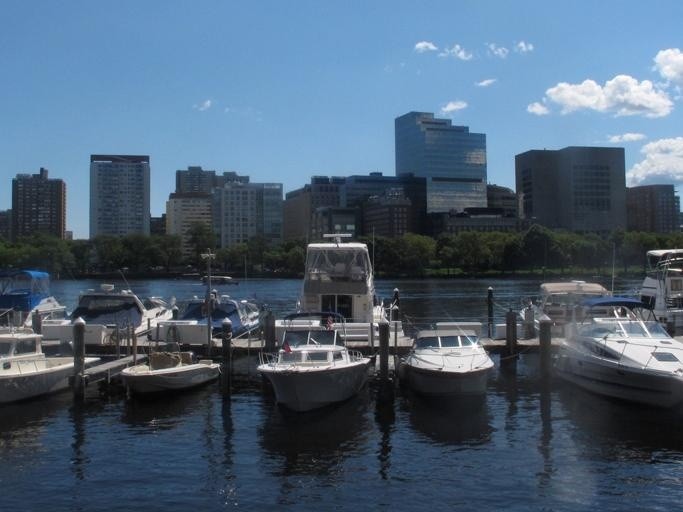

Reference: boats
[40,282,174,345]
[549,296,683,411]
[519,280,622,337]
[633,248,683,334]
[0,268,54,332]
[298,241,389,333]
[119,321,220,393]
[0,329,101,405]
[148,246,265,341]
[255,311,373,413]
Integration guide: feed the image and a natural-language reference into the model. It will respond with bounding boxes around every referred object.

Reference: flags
[324,316,332,330]
[282,338,292,352]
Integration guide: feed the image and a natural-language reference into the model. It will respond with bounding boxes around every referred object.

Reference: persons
[663,317,673,337]
[657,316,667,328]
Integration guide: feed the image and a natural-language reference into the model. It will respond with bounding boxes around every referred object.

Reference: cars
[398,327,495,398]
